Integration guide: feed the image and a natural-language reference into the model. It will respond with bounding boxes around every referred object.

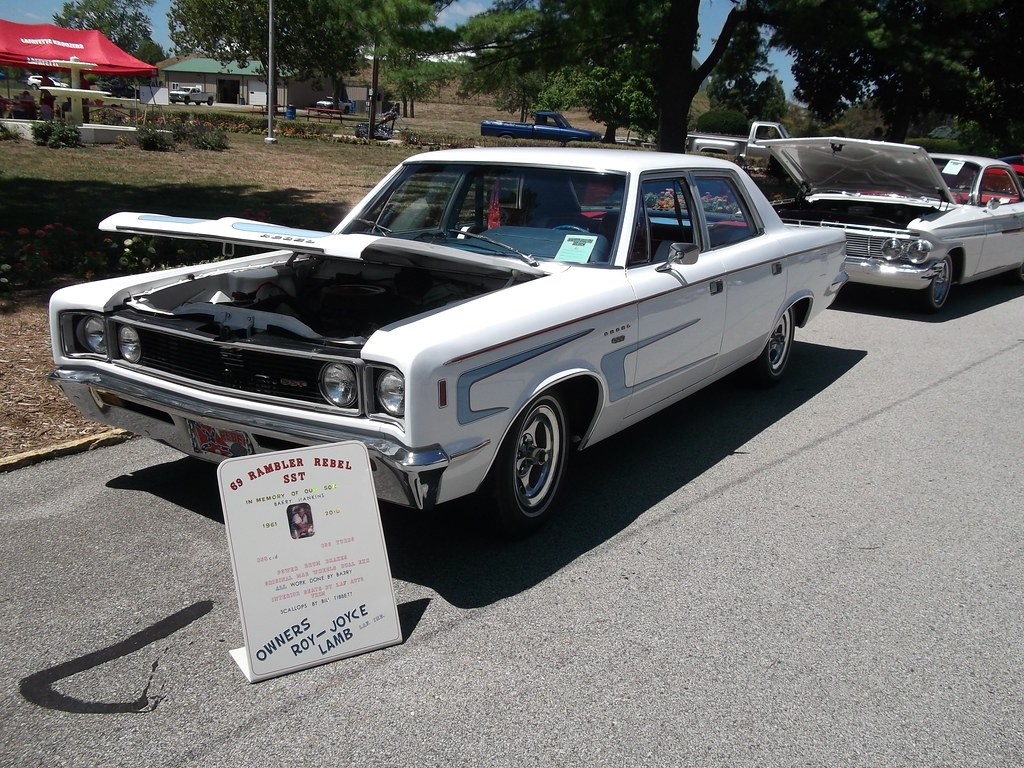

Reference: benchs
[249,104,278,118]
[305,107,342,124]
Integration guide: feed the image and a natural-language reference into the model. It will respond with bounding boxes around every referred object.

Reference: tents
[0,19,160,133]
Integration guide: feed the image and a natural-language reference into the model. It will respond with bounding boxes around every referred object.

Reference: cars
[47,145,854,534]
[761,135,1024,311]
[954,153,1024,206]
[27,76,70,90]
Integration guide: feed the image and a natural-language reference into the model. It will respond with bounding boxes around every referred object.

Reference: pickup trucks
[684,120,790,156]
[480,111,601,145]
[316,96,351,114]
[169,87,217,106]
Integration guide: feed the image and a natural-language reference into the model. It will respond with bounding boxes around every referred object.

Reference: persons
[6,67,90,126]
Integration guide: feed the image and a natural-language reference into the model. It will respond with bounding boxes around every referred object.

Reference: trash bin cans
[239,98,244,104]
[285,105,296,120]
